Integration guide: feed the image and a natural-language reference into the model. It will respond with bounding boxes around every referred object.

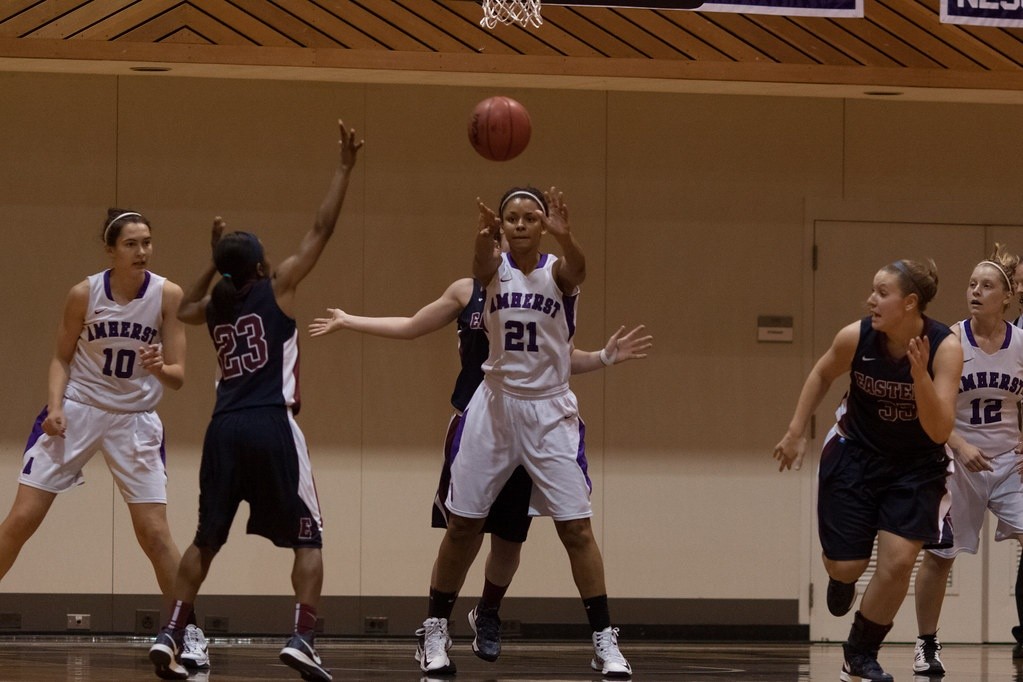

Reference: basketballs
[466,94,533,163]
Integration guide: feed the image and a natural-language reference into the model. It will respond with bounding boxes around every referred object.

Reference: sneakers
[590,626,633,682]
[839,642,894,682]
[419,674,450,682]
[148,627,189,680]
[1012,625,1023,660]
[413,617,457,678]
[179,624,210,669]
[186,664,211,682]
[911,628,946,674]
[278,633,333,682]
[826,576,857,617]
[467,603,501,662]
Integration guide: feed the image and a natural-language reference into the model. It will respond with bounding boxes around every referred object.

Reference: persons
[772,241,1023,682]
[0,117,655,679]
